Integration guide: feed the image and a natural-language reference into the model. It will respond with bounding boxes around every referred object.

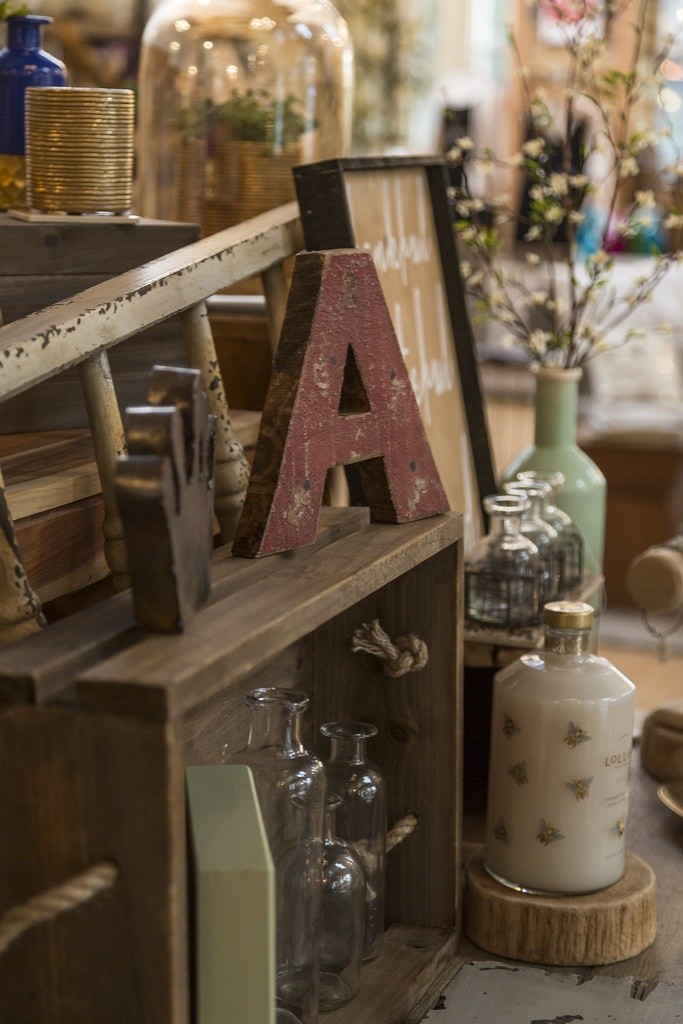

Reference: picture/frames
[289,152,501,555]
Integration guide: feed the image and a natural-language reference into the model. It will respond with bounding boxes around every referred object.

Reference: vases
[501,365,607,655]
[0,11,71,157]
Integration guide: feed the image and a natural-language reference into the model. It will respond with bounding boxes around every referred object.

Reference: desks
[0,506,465,1024]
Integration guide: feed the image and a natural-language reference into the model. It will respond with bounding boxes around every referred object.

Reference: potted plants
[163,93,325,296]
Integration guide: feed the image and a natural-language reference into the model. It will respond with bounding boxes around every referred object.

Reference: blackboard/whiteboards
[291,154,499,549]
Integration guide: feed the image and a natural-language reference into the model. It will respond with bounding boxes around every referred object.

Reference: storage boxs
[184,765,276,1024]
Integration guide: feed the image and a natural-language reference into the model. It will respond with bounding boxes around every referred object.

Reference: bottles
[277,793,365,1012]
[136,0,354,224]
[465,469,585,625]
[228,687,326,1024]
[484,601,636,896]
[318,719,388,964]
[0,14,68,154]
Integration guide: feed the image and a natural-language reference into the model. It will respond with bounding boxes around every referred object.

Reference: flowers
[438,0,683,371]
